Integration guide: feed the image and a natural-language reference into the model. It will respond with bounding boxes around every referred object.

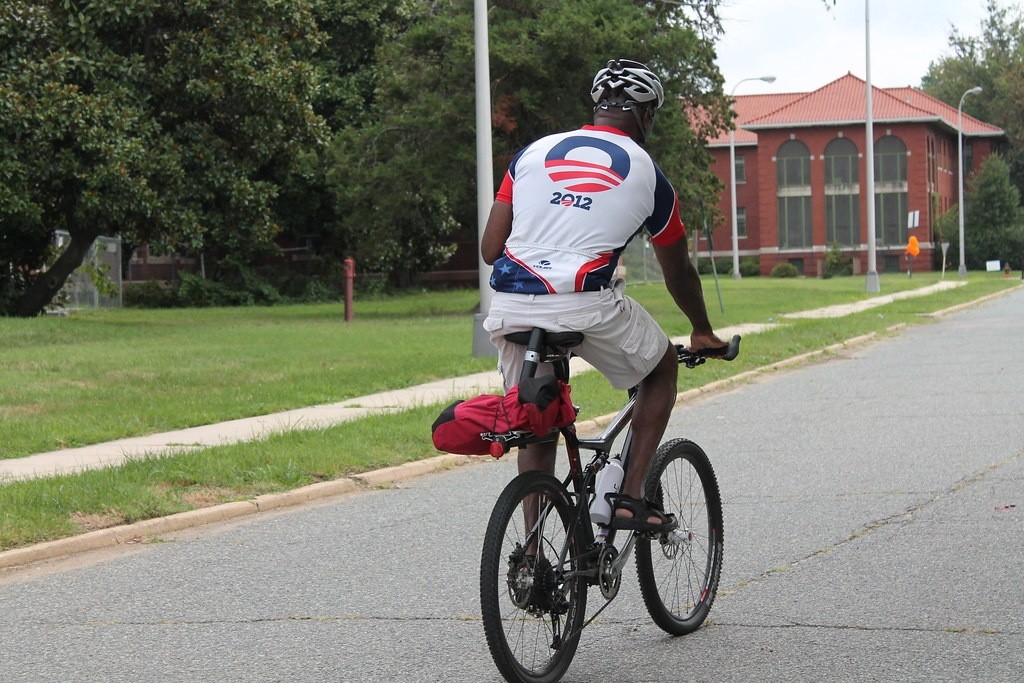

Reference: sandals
[520,555,569,613]
[612,494,678,532]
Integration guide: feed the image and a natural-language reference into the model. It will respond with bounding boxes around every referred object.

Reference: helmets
[590,59,664,110]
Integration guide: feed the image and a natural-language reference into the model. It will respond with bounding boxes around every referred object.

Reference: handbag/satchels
[432,375,576,455]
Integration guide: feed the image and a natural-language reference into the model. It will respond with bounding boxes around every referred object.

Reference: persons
[481,56,730,577]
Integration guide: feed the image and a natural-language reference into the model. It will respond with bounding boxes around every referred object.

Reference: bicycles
[478,326,741,683]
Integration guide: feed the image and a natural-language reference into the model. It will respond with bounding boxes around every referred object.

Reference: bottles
[589,454,624,524]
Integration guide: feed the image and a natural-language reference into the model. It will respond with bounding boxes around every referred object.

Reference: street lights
[957,87,983,277]
[727,76,777,281]
